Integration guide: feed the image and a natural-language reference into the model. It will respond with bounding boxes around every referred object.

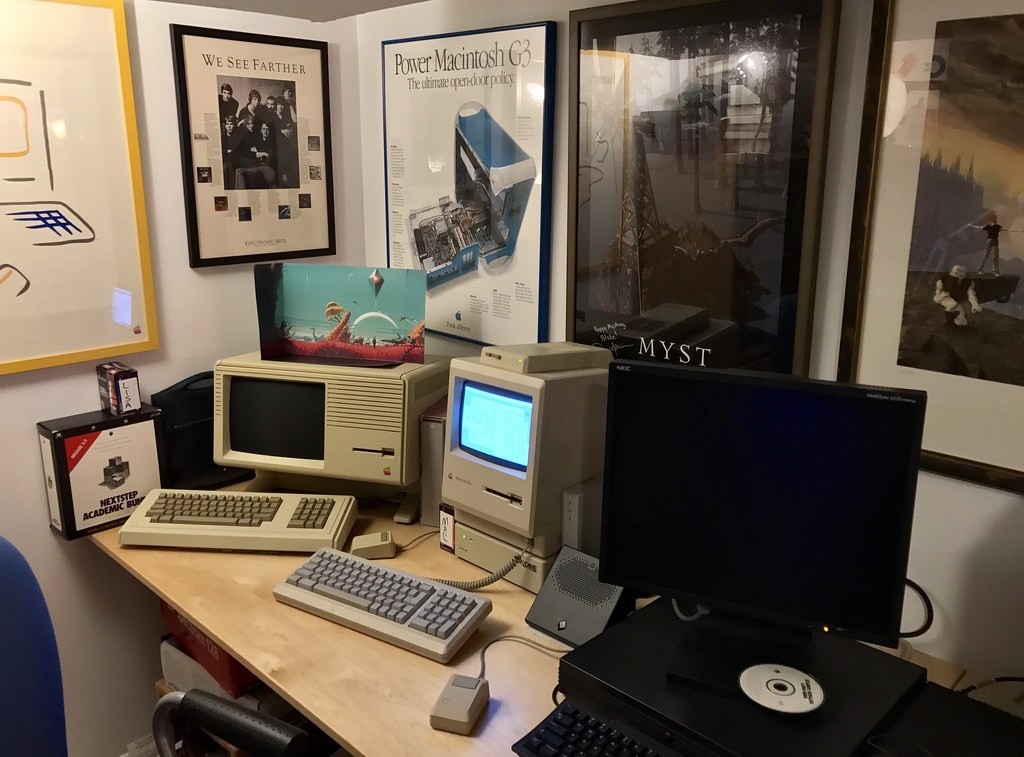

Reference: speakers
[152,371,256,491]
[525,544,634,649]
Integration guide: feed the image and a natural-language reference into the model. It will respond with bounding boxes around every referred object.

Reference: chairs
[0,535,311,757]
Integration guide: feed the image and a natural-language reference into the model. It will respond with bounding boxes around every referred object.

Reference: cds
[739,663,824,713]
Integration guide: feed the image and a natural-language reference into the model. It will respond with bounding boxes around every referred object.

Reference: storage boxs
[36,402,170,541]
[159,599,259,700]
[153,676,309,757]
[96,360,142,419]
[160,633,295,718]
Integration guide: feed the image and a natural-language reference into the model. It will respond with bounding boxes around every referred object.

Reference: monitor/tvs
[214,351,454,524]
[441,354,609,559]
[599,358,929,709]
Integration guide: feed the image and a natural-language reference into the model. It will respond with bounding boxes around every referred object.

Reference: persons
[968,215,1023,273]
[218,82,301,190]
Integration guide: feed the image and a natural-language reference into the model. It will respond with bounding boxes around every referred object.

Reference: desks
[86,475,967,757]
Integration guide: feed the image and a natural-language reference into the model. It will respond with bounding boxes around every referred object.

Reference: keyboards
[118,489,358,553]
[274,546,493,664]
[510,691,682,757]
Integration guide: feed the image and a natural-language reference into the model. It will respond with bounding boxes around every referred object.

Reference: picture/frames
[0,0,159,376]
[565,0,896,381]
[836,0,1024,496]
[169,23,336,268]
